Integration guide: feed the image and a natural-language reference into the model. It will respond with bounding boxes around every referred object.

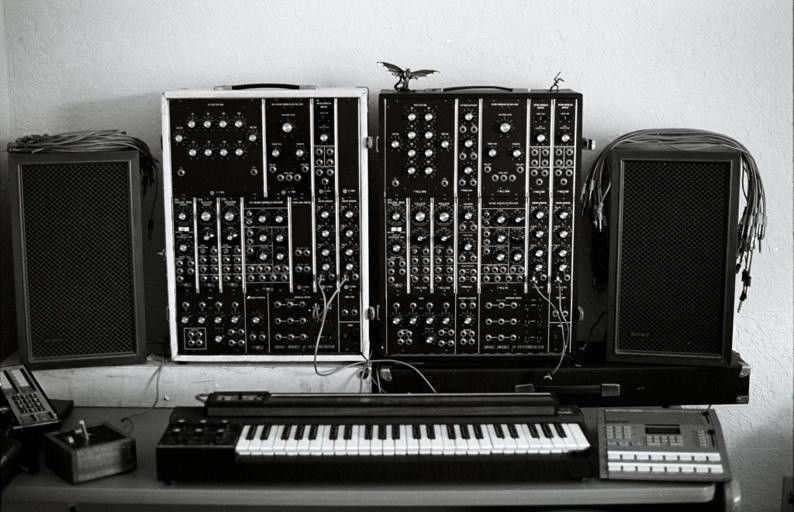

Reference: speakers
[6,148,148,372]
[606,145,741,368]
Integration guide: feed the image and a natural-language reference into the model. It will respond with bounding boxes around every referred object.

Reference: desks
[0,396,744,512]
